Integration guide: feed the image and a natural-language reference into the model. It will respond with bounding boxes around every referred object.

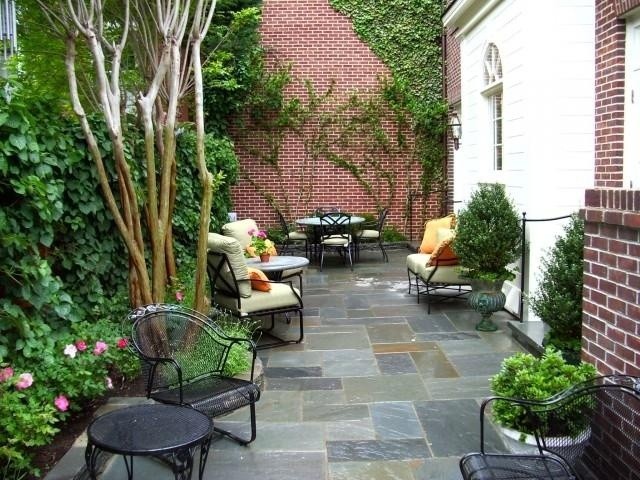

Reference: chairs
[208,231,303,351]
[459,374,640,480]
[406,228,475,315]
[121,302,260,471]
[276,206,390,271]
[221,219,302,299]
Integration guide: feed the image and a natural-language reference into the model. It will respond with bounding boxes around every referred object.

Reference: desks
[85,404,213,480]
[245,255,310,324]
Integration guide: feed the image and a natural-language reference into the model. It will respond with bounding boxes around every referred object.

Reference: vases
[260,252,270,262]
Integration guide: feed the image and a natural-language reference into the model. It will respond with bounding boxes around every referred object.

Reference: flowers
[248,229,272,256]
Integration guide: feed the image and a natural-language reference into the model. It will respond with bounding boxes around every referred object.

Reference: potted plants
[528,207,585,367]
[488,343,601,476]
[452,182,529,332]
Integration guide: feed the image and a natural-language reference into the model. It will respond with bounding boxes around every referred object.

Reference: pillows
[417,212,455,253]
[437,228,455,244]
[246,240,277,255]
[248,267,272,291]
[425,234,463,268]
